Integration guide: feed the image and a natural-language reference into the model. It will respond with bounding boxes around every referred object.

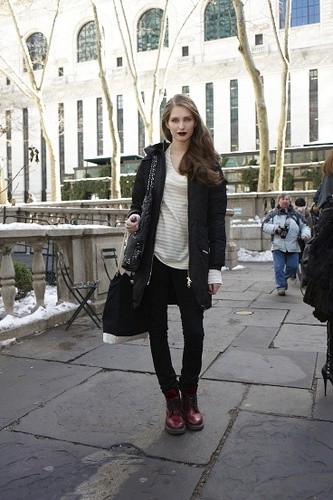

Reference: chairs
[58,250,102,331]
[100,248,119,282]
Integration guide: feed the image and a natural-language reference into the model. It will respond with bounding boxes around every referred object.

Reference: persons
[125,94,227,435]
[260,151,333,396]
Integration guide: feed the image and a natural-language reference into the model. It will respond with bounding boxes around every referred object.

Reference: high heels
[321,322,333,397]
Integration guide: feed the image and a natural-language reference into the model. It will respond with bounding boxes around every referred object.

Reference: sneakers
[276,287,286,296]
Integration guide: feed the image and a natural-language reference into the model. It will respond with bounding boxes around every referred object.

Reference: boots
[182,380,205,432]
[163,388,186,435]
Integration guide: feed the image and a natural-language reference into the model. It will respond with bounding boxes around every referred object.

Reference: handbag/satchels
[99,272,150,347]
